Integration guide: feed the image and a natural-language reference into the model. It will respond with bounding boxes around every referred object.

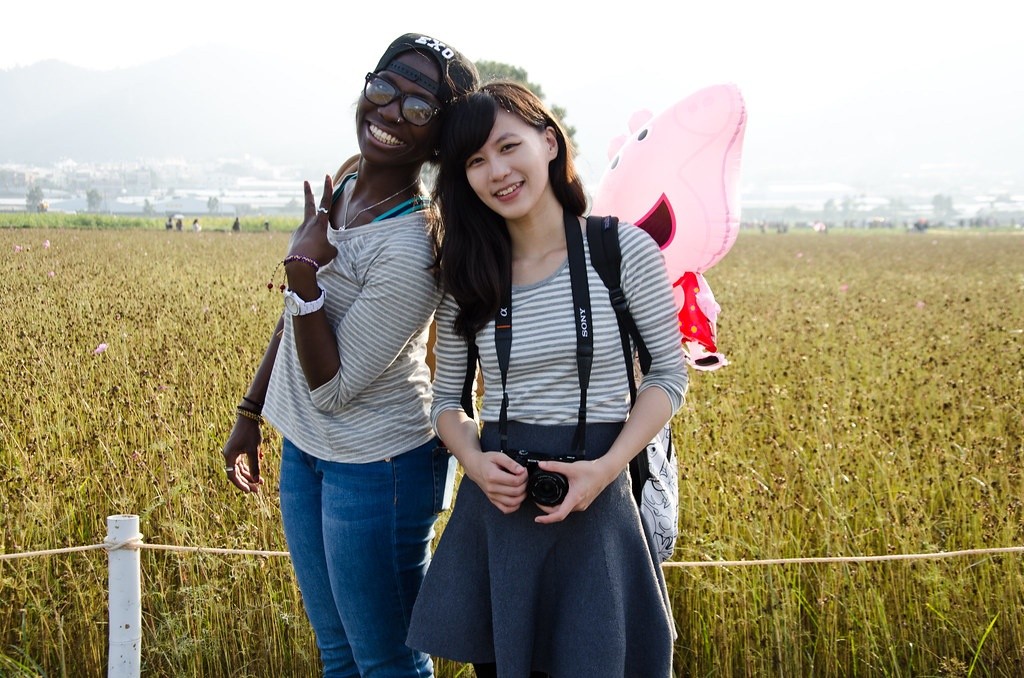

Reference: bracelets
[237,397,265,425]
[268,256,319,293]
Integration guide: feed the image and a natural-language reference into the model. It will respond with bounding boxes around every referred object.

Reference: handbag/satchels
[627,418,680,563]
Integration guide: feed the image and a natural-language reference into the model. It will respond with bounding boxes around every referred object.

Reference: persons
[417,109,431,121]
[224,33,480,678]
[405,82,690,678]
[166,215,269,232]
[759,215,1024,233]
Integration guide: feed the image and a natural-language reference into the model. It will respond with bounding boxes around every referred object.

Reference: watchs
[285,288,326,316]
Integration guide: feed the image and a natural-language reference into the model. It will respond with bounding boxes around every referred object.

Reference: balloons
[593,83,748,371]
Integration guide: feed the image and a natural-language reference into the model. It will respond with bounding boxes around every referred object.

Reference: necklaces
[339,177,419,231]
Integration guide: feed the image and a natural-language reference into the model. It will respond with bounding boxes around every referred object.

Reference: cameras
[511,450,578,507]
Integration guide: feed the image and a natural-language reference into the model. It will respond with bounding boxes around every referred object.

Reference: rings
[318,208,329,214]
[225,466,238,471]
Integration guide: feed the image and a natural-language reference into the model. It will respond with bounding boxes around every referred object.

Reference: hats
[373,32,480,105]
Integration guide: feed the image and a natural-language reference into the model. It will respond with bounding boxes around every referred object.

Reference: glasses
[364,73,442,127]
